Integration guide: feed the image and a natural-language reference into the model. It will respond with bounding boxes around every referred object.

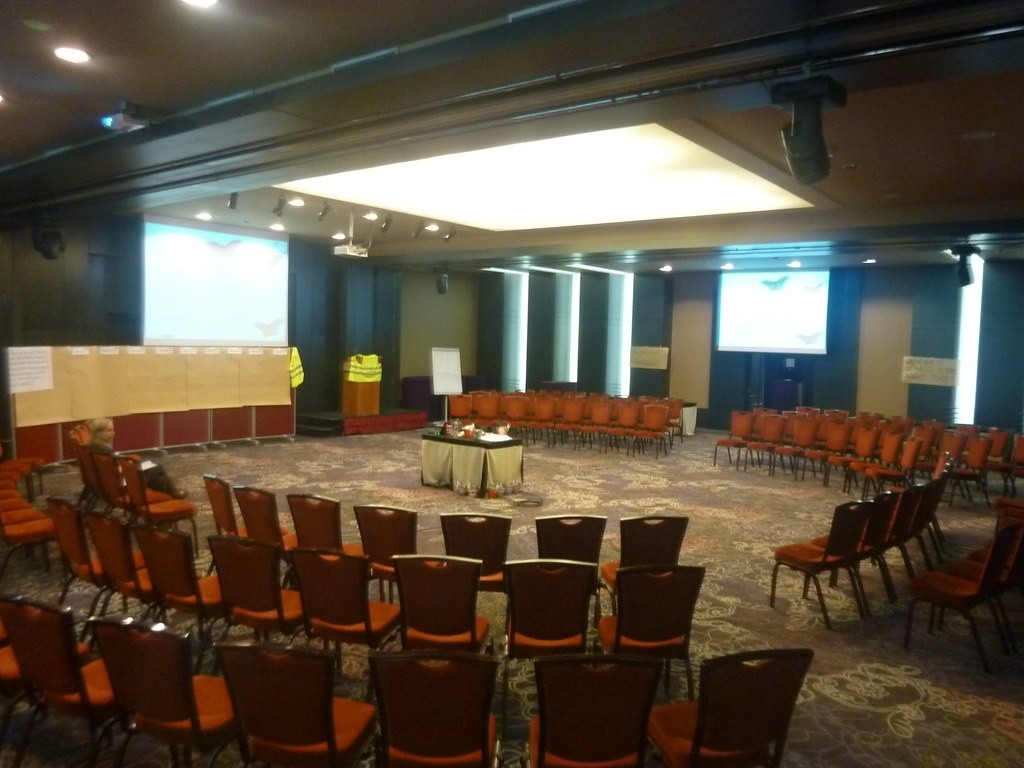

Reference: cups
[498,426,509,436]
[473,429,481,440]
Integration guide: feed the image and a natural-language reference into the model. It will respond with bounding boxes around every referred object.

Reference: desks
[421,431,524,499]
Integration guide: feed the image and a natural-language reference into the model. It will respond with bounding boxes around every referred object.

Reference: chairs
[647,645,815,768]
[768,482,942,630]
[0,425,707,768]
[903,497,1024,676]
[712,406,1024,498]
[451,391,683,460]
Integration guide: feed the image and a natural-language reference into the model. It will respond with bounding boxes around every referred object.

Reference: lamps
[382,215,392,233]
[31,226,67,259]
[952,244,980,288]
[273,195,287,217]
[443,227,457,244]
[413,221,426,239]
[770,77,848,188]
[317,204,332,222]
[437,264,449,292]
[228,192,239,210]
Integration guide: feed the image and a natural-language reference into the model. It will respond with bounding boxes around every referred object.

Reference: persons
[90,417,188,500]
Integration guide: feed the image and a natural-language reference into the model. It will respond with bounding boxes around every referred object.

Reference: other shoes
[176,489,189,500]
